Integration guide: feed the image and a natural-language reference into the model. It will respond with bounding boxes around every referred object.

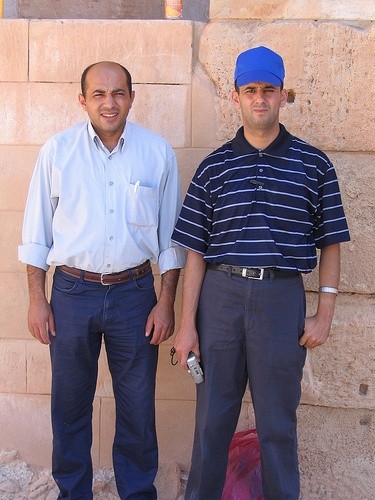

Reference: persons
[171,45,350,500]
[19,60,188,500]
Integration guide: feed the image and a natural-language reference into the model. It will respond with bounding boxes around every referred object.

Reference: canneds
[164,0,183,20]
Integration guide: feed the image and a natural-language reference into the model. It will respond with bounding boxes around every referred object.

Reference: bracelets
[319,287,338,296]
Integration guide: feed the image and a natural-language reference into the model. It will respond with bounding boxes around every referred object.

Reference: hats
[234,46,285,87]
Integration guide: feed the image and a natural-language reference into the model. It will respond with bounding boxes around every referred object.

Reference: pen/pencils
[133,180,140,194]
[249,180,266,185]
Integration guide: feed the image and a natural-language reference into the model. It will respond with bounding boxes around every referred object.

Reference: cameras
[186,352,204,385]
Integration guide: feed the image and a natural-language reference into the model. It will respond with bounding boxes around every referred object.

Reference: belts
[206,262,301,281]
[56,262,152,285]
